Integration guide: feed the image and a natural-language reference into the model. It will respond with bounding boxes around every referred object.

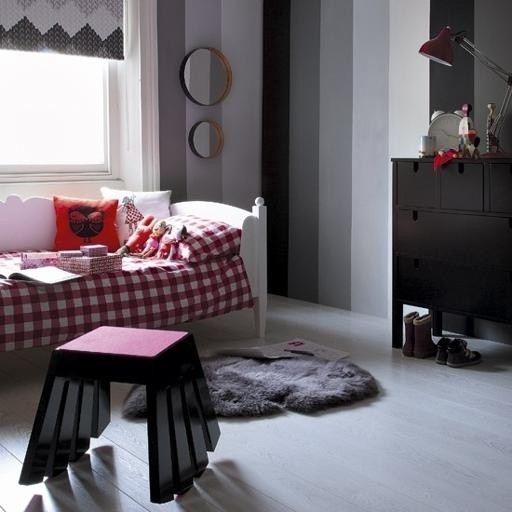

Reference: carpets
[121,350,378,419]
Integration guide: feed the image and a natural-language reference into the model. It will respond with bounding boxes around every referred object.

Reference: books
[0,265,82,285]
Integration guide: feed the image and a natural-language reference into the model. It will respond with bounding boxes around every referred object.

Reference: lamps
[418,25,510,160]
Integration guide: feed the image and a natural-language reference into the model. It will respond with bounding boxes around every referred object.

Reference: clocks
[426,111,471,156]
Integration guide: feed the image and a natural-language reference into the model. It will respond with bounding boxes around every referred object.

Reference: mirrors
[178,43,232,161]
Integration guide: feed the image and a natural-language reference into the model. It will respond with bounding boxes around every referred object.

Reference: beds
[1,189,266,354]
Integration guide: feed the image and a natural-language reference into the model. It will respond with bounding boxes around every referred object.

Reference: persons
[155,223,188,260]
[137,219,167,259]
[113,215,153,258]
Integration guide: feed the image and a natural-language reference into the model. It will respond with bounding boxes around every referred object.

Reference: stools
[19,327,223,504]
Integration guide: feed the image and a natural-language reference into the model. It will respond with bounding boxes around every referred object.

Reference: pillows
[53,186,243,262]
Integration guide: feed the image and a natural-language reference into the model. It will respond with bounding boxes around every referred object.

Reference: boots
[414,314,438,358]
[403,312,419,357]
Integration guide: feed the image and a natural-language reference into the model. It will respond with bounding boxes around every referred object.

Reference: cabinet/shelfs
[392,158,511,348]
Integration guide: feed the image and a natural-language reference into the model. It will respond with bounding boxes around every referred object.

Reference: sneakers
[447,339,481,368]
[436,338,451,365]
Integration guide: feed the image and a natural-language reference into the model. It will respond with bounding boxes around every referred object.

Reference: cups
[420,136,436,157]
